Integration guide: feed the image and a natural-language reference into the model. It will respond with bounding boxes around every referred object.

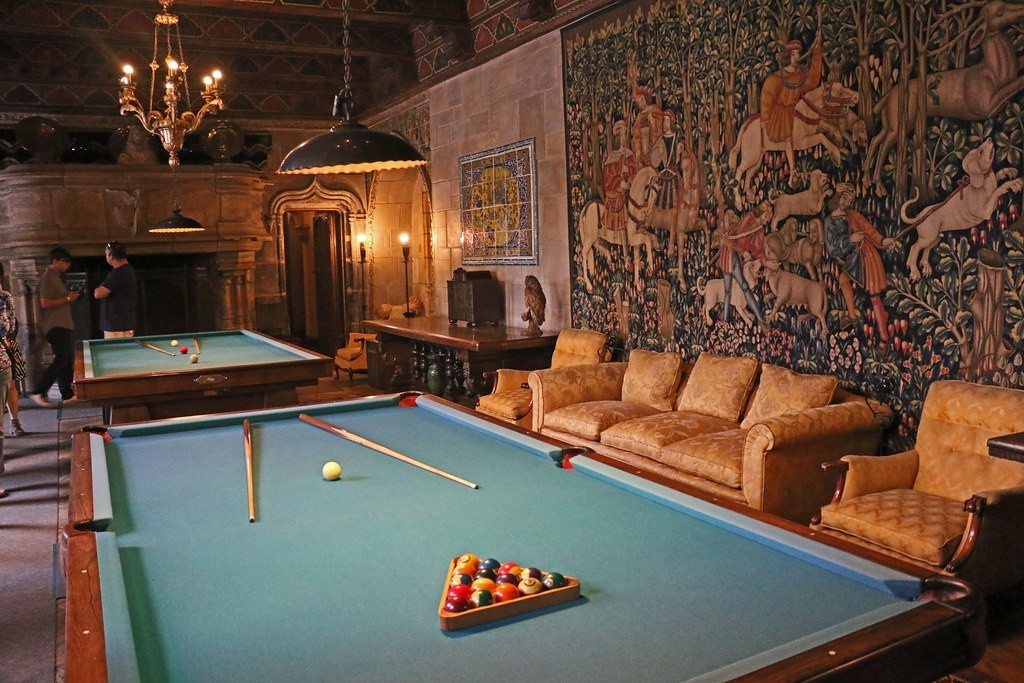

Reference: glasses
[54,258,72,265]
[107,240,118,252]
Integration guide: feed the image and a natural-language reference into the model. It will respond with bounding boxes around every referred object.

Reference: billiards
[170,340,178,347]
[190,354,198,362]
[443,552,566,612]
[322,461,342,481]
[180,346,188,354]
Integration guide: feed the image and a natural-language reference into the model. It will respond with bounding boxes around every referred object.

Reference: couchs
[335,297,424,386]
[811,379,1024,603]
[526,362,892,525]
[474,328,625,428]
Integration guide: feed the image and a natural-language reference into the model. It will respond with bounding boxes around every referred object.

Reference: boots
[10,419,24,435]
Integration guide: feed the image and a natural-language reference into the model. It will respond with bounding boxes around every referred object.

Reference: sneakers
[29,394,54,408]
[62,395,83,405]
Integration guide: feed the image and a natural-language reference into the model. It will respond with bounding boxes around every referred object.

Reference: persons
[0,265,26,436]
[27,247,88,407]
[94,240,138,338]
[0,265,16,500]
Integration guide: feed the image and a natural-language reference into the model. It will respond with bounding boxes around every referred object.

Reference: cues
[134,339,176,357]
[193,336,200,354]
[243,417,255,523]
[299,412,479,490]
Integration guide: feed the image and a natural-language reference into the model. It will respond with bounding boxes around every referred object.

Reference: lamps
[358,235,368,333]
[119,0,227,169]
[148,171,205,232]
[273,0,431,174]
[400,233,417,318]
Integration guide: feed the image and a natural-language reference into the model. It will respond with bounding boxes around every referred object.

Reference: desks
[61,390,984,683]
[73,328,334,426]
[362,316,560,414]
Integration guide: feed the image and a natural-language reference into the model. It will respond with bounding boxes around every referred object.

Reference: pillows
[621,349,682,412]
[679,352,757,422]
[740,363,838,429]
